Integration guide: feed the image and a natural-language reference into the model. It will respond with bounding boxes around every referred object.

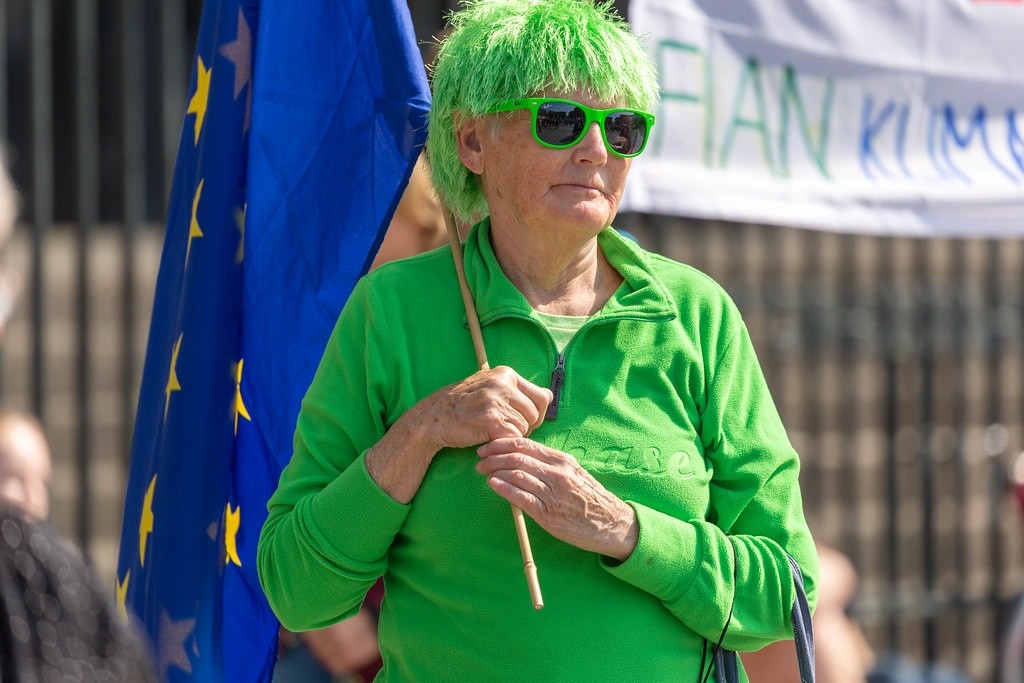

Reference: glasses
[461,90,658,161]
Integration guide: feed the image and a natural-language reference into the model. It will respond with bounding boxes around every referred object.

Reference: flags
[116,1,435,683]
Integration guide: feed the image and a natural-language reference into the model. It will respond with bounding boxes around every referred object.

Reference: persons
[255,0,823,682]
